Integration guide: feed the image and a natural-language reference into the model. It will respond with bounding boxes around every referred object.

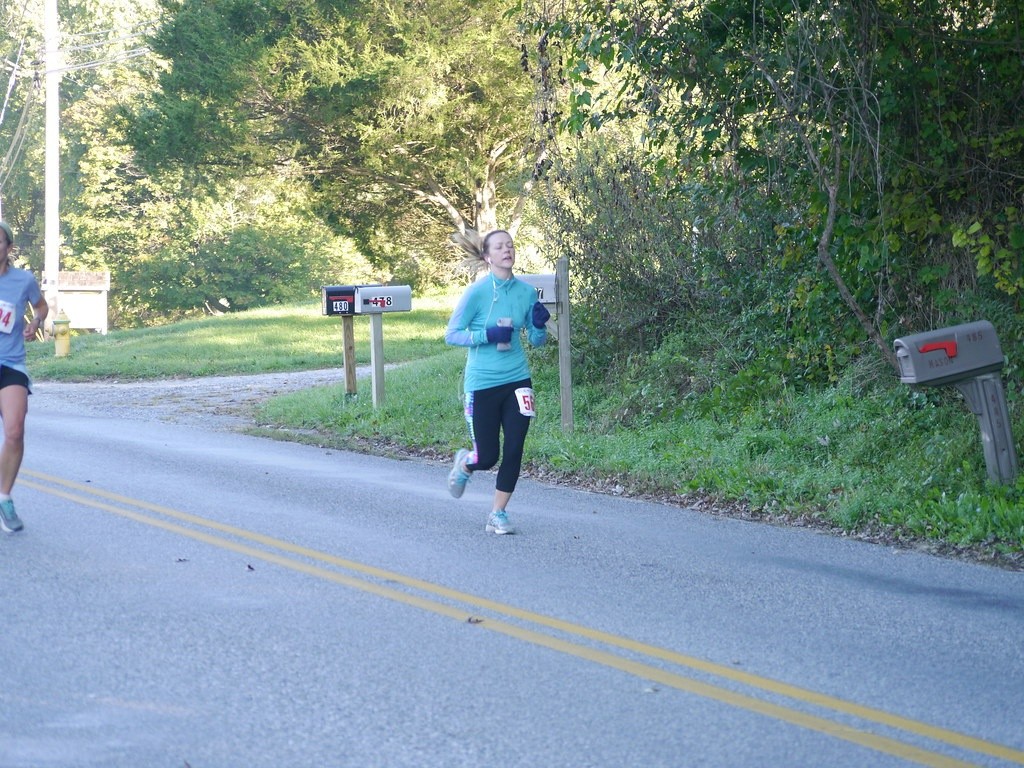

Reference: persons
[445,230,550,534]
[0,222,49,532]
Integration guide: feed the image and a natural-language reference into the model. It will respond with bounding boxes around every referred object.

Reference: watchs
[36,317,42,328]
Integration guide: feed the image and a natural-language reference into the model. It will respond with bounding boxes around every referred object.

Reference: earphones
[488,257,491,264]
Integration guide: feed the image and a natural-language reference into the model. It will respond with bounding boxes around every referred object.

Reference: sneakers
[0,499,23,533]
[486,509,514,535]
[448,449,476,498]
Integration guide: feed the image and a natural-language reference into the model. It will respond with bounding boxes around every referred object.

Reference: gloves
[486,325,514,343]
[532,301,550,329]
